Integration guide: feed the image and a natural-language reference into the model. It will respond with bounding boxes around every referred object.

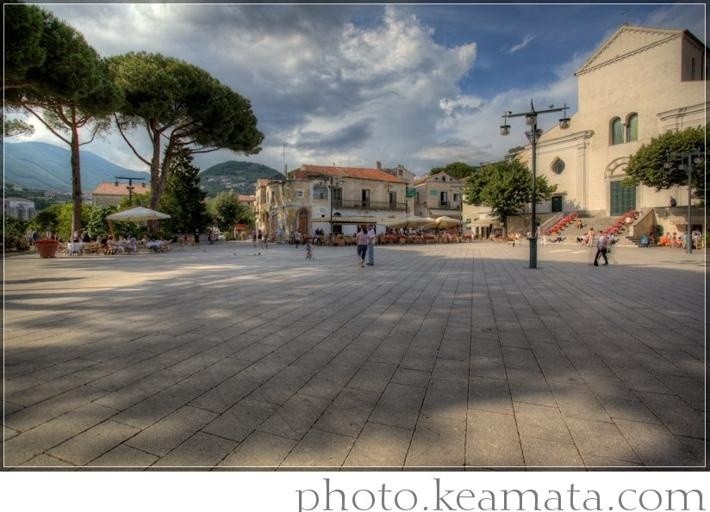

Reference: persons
[355,225,371,268]
[644,228,702,250]
[251,224,476,249]
[593,230,608,266]
[575,219,582,228]
[193,228,201,249]
[670,196,678,207]
[365,225,376,266]
[303,240,312,258]
[208,230,213,245]
[574,227,614,247]
[25,224,174,255]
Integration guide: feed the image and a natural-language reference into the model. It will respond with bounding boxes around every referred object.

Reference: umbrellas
[380,214,463,228]
[105,206,172,222]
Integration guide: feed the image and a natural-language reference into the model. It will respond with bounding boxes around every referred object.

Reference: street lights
[662,146,705,256]
[112,172,147,239]
[500,101,570,269]
